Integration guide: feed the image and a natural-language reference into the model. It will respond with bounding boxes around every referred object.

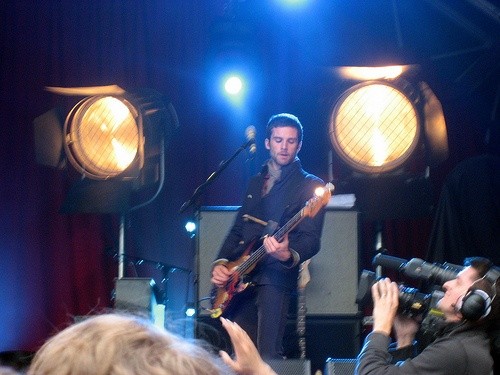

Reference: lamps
[49,81,181,187]
[324,62,453,182]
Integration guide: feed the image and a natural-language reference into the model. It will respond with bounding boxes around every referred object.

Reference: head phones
[454,266,499,320]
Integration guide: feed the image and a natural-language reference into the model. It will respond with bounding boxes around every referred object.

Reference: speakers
[194,206,361,317]
[192,317,359,375]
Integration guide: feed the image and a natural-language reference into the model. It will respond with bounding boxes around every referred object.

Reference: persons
[355,257,500,375]
[25,313,279,375]
[210,113,328,360]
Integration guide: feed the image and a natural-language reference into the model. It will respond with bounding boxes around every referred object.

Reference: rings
[380,294,386,296]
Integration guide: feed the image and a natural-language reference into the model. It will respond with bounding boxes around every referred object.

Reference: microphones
[246,125,256,152]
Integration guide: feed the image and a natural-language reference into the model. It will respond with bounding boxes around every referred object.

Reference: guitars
[211,180,335,316]
